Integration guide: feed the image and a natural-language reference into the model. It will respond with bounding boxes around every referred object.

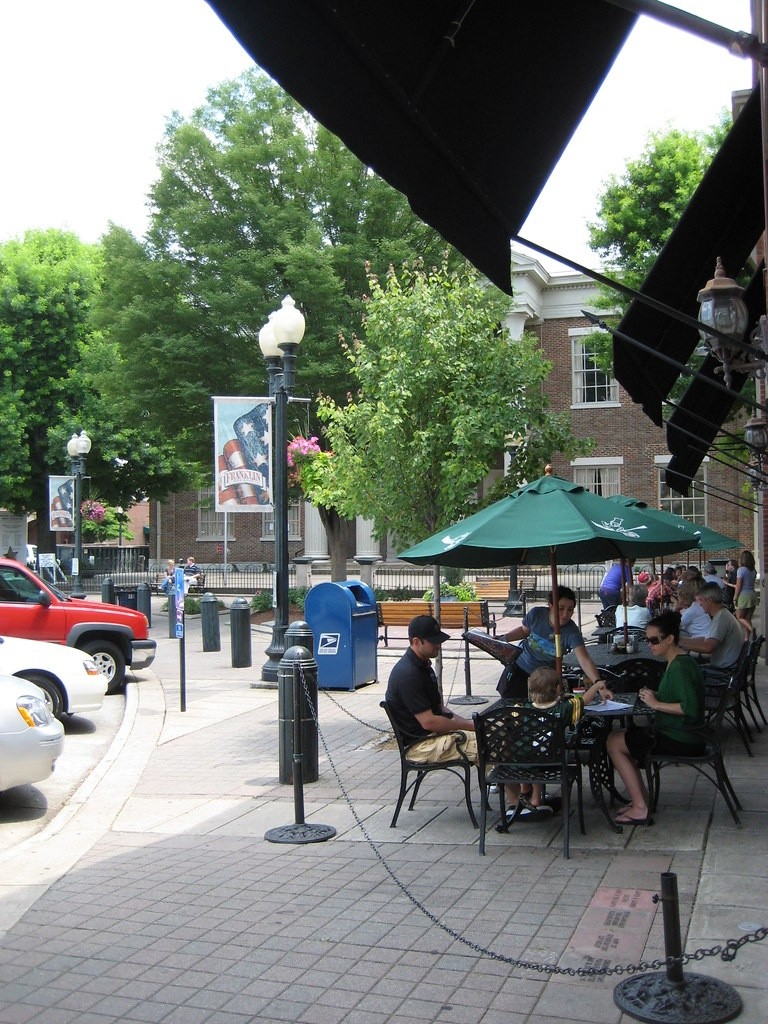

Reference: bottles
[626,641,634,654]
[611,643,617,654]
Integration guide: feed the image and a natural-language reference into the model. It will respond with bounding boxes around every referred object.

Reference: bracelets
[595,679,601,684]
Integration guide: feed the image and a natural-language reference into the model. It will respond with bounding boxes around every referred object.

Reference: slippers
[615,804,654,826]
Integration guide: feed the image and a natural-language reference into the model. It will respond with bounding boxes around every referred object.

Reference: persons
[607,611,709,824]
[385,615,562,821]
[599,550,757,687]
[159,557,201,597]
[512,666,606,807]
[487,585,615,793]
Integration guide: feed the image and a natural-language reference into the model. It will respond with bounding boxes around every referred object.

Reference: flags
[214,399,273,513]
[49,478,74,531]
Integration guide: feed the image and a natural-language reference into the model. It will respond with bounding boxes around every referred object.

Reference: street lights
[505,430,524,619]
[118,506,123,544]
[69,429,93,600]
[254,297,305,679]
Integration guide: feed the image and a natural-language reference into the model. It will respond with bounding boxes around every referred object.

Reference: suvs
[0,558,156,695]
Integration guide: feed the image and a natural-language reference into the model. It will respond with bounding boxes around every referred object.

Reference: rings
[641,695,644,699]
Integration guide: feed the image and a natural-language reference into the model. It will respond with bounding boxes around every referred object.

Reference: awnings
[206,0,651,298]
[612,81,768,499]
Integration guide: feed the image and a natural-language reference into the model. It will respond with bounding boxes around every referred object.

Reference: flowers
[80,489,106,524]
[289,422,340,495]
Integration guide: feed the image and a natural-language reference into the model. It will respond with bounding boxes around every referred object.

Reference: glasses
[645,635,668,645]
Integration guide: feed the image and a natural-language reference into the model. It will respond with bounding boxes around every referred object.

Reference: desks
[479,691,638,835]
[591,626,616,644]
[562,641,689,724]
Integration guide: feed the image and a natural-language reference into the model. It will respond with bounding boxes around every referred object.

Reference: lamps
[744,457,768,494]
[741,406,768,472]
[696,255,768,390]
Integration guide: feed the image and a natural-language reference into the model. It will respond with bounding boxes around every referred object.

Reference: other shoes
[490,782,499,793]
[751,627,756,641]
[505,804,554,822]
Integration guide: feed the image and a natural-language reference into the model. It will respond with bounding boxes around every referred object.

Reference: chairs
[379,700,495,829]
[471,706,588,861]
[563,589,768,831]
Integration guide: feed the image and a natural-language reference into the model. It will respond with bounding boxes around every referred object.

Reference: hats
[408,615,451,645]
[702,563,715,574]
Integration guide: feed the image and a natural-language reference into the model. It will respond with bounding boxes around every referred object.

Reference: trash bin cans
[305,580,381,690]
[114,584,138,610]
[708,559,729,579]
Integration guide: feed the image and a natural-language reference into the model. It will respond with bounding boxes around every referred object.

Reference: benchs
[476,575,538,602]
[375,601,497,648]
[148,572,207,597]
[474,580,524,603]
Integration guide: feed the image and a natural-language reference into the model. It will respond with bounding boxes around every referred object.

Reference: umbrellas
[395,464,747,693]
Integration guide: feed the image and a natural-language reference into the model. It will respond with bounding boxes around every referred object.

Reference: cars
[0,635,109,721]
[0,676,67,791]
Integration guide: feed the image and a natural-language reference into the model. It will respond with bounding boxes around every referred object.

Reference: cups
[572,687,585,695]
